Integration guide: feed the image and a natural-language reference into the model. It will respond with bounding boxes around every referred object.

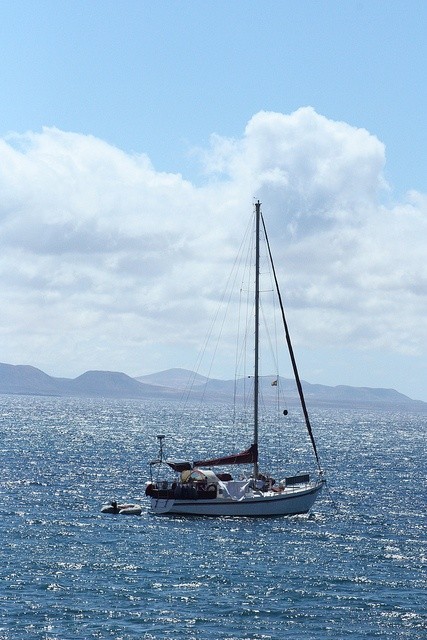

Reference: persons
[108,501,121,514]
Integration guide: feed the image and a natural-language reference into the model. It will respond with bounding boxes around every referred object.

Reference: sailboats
[146,193,327,516]
